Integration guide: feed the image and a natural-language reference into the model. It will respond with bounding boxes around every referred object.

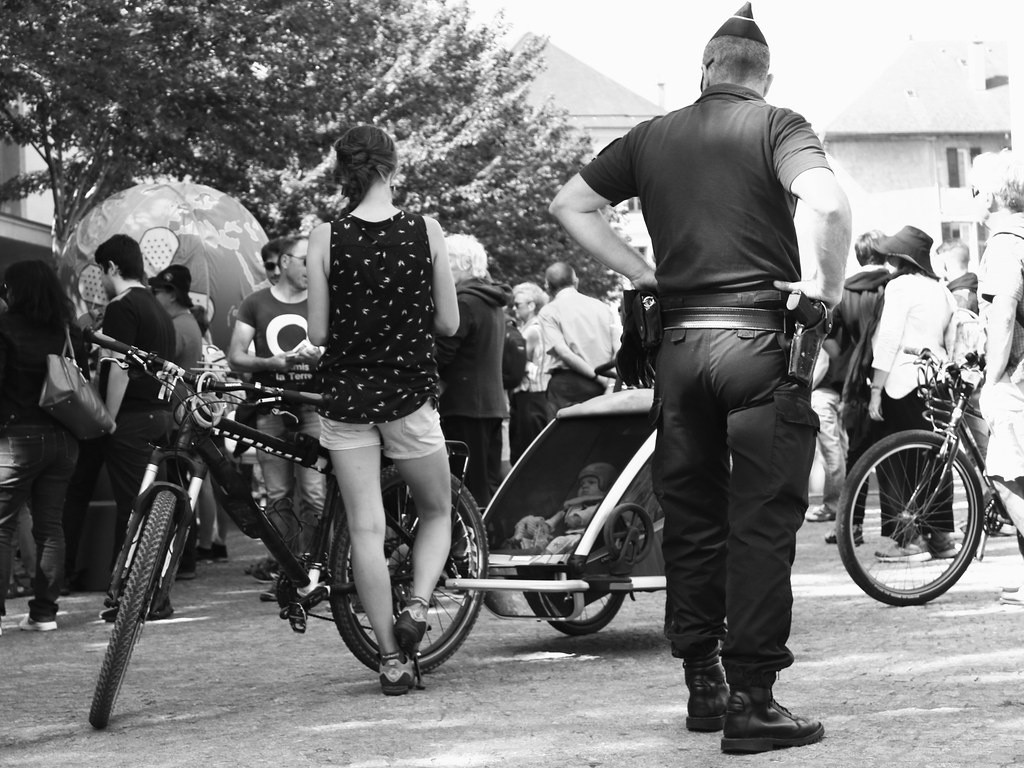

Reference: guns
[785,286,830,380]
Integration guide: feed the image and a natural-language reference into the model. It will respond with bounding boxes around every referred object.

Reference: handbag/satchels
[37,321,115,441]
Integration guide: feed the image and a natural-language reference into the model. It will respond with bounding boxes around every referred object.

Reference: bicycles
[836,344,1024,607]
[77,323,490,731]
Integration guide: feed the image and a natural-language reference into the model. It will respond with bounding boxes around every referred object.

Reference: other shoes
[194,545,216,564]
[61,585,71,598]
[98,596,163,623]
[18,612,59,631]
[873,535,933,564]
[176,559,198,581]
[7,583,34,599]
[259,581,279,600]
[1002,588,1024,606]
[928,533,964,556]
[212,544,229,563]
[825,525,864,545]
[806,503,841,523]
[251,562,283,584]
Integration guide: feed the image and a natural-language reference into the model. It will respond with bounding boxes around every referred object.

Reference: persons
[227,235,624,601]
[0,234,229,631]
[307,126,460,694]
[805,145,1024,605]
[547,2,852,754]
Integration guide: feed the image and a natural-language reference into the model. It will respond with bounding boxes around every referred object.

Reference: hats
[876,225,941,281]
[147,264,194,308]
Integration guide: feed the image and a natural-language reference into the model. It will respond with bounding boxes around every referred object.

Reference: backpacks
[500,318,528,393]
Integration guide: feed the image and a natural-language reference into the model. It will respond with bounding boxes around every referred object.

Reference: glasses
[263,262,279,272]
[287,253,306,266]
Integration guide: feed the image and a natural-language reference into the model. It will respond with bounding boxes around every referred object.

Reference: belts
[656,303,787,334]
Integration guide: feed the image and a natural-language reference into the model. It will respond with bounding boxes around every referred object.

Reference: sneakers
[378,655,419,695]
[397,611,428,653]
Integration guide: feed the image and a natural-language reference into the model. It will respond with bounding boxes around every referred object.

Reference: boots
[720,681,825,755]
[682,649,726,734]
